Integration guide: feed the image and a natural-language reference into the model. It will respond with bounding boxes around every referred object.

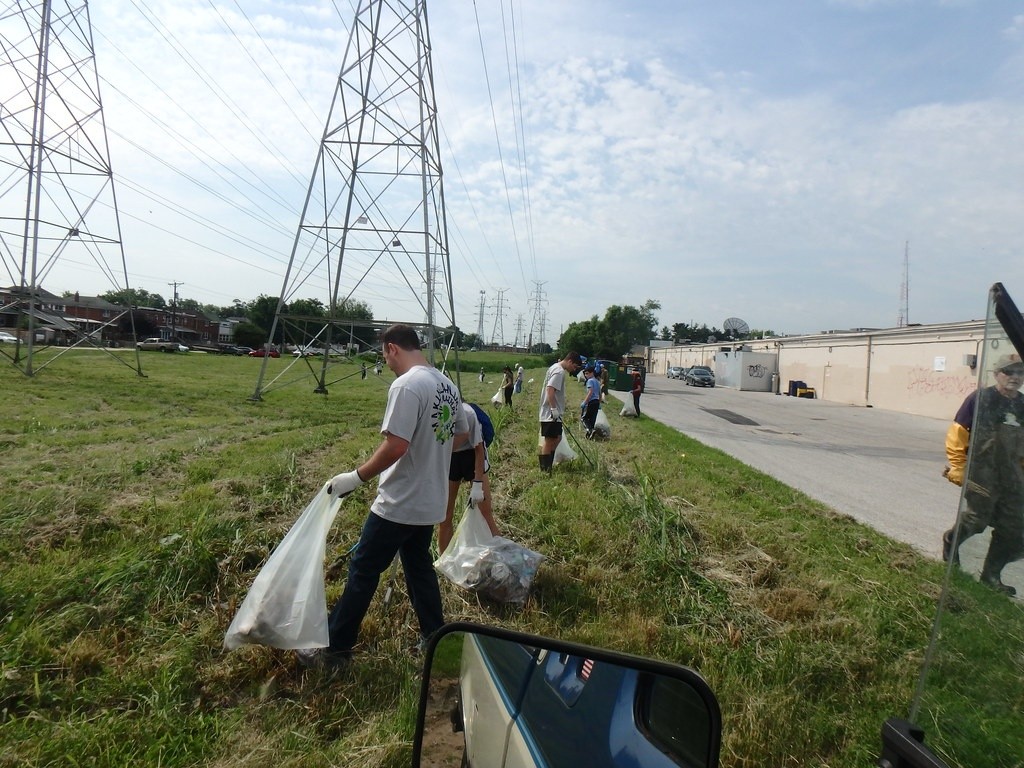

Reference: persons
[361,362,368,381]
[515,362,524,393]
[480,367,485,381]
[594,357,601,377]
[377,361,384,375]
[584,360,590,368]
[294,323,468,675]
[631,368,643,417]
[502,366,514,407]
[943,351,1024,595]
[580,366,602,440]
[537,351,582,477]
[599,363,610,396]
[436,403,502,559]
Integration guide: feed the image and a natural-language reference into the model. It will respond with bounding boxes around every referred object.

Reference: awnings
[164,325,196,333]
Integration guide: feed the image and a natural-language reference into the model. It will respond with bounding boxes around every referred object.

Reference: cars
[666,365,715,380]
[684,369,715,388]
[238,347,254,354]
[441,342,479,352]
[172,343,190,352]
[249,348,281,359]
[447,632,715,768]
[279,342,349,357]
[0,331,24,345]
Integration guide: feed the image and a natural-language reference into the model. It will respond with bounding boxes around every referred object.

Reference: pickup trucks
[206,343,245,357]
[136,338,179,354]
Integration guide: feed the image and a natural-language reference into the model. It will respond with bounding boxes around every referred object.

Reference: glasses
[1000,367,1024,376]
[504,370,508,374]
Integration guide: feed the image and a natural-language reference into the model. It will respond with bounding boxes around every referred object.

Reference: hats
[515,363,519,368]
[992,353,1024,370]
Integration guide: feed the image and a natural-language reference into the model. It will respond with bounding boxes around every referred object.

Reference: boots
[943,515,974,567]
[980,535,1016,595]
[539,451,555,475]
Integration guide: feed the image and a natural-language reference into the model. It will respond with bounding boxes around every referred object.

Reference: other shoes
[295,646,321,666]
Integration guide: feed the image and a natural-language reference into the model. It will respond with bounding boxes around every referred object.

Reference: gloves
[467,480,485,509]
[328,469,367,498]
[550,407,560,422]
[581,402,587,408]
[944,421,969,487]
